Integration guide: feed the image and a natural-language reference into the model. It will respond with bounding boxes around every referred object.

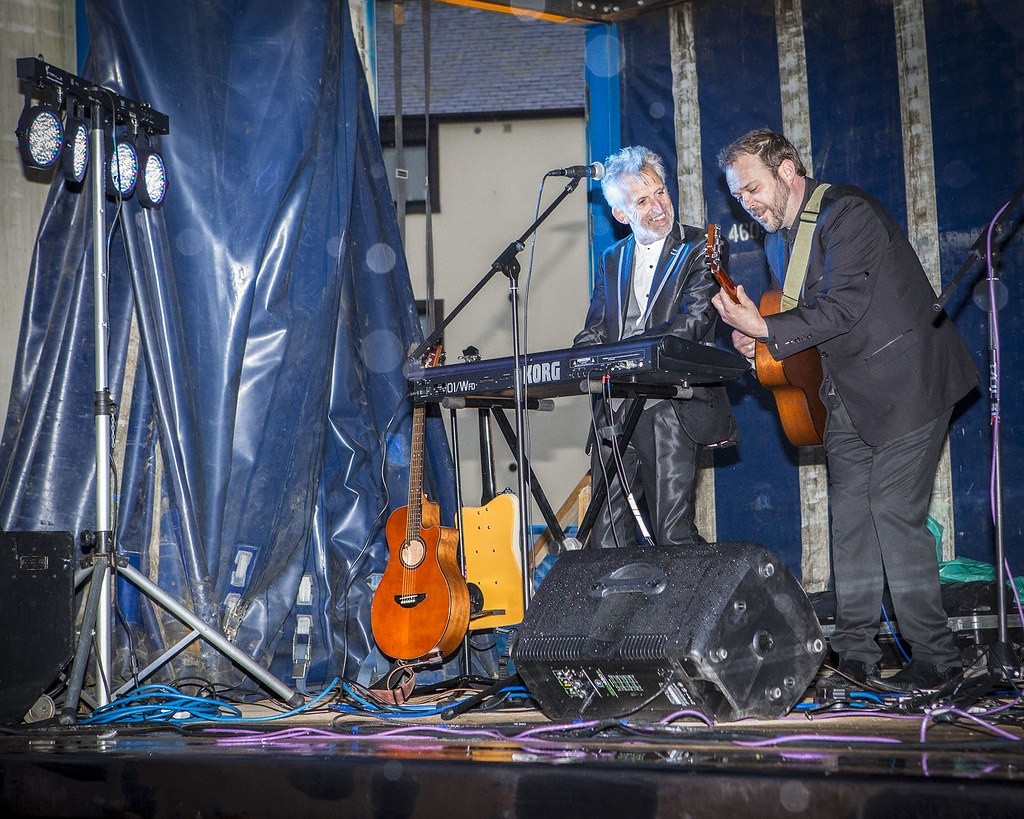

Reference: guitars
[370,341,471,661]
[705,218,825,444]
[453,343,534,632]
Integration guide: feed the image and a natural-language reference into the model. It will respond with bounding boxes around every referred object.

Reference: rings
[748,343,754,351]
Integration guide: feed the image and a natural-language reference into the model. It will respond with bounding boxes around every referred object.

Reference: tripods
[58,104,306,727]
[899,191,1024,727]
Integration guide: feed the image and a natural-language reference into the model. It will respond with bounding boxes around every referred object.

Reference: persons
[711,129,982,695]
[572,145,731,549]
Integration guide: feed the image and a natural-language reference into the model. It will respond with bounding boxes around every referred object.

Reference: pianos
[408,331,748,403]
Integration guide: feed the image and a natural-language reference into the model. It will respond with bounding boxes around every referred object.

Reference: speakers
[511,541,827,726]
[0,530,76,730]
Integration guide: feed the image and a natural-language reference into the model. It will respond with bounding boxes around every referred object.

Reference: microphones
[549,161,605,180]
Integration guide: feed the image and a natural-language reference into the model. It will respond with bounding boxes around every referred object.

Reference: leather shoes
[816,659,870,692]
[866,656,963,694]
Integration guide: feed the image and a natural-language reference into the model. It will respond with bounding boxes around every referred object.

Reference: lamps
[15,106,63,169]
[105,135,140,198]
[60,118,90,183]
[138,148,169,209]
